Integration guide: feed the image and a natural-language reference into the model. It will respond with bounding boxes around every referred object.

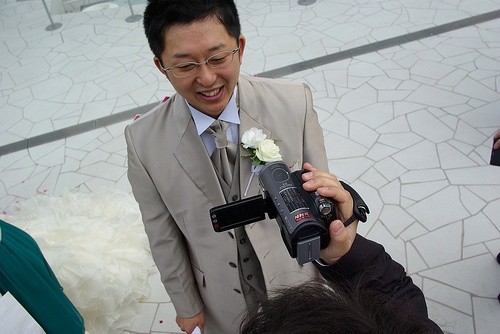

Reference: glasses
[159,36,240,78]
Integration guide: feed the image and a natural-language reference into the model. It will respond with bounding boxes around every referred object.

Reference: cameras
[209,161,336,264]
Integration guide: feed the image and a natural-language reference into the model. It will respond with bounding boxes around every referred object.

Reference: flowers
[239,127,283,197]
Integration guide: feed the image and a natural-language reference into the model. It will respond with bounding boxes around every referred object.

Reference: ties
[206,120,237,187]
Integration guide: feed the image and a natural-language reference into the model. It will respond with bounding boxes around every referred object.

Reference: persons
[237,162,444,334]
[124,0,330,334]
